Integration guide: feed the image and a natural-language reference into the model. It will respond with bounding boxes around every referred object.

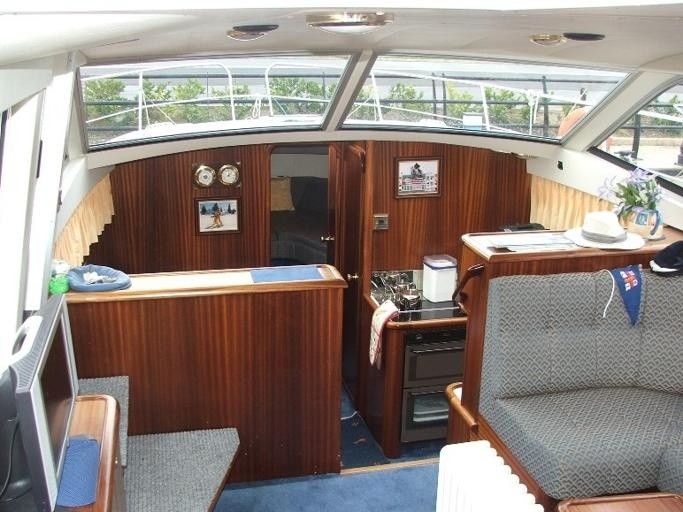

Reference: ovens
[398,381,454,442]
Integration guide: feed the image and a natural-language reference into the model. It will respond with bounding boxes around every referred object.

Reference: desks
[54,394,127,512]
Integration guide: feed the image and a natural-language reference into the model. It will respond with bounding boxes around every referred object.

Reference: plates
[581,228,628,243]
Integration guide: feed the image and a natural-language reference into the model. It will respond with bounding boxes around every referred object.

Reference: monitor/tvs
[0,292,81,512]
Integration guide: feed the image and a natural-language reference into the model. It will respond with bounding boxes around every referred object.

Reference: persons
[214,208,223,227]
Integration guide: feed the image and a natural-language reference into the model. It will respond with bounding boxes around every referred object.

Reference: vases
[596,167,660,211]
[616,206,665,241]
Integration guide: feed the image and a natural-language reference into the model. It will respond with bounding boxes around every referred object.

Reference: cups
[372,268,420,313]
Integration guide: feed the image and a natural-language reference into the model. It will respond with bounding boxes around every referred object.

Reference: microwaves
[402,329,471,389]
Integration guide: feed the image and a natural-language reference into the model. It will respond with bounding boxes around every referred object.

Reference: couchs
[475,267,683,511]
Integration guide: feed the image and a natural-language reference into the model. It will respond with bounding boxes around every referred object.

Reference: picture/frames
[194,197,242,234]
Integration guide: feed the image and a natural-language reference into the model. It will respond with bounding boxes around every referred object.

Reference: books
[486,235,576,252]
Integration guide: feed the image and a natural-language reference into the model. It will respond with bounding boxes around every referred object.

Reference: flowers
[393,156,442,199]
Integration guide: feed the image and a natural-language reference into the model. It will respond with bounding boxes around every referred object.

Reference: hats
[564,212,644,250]
